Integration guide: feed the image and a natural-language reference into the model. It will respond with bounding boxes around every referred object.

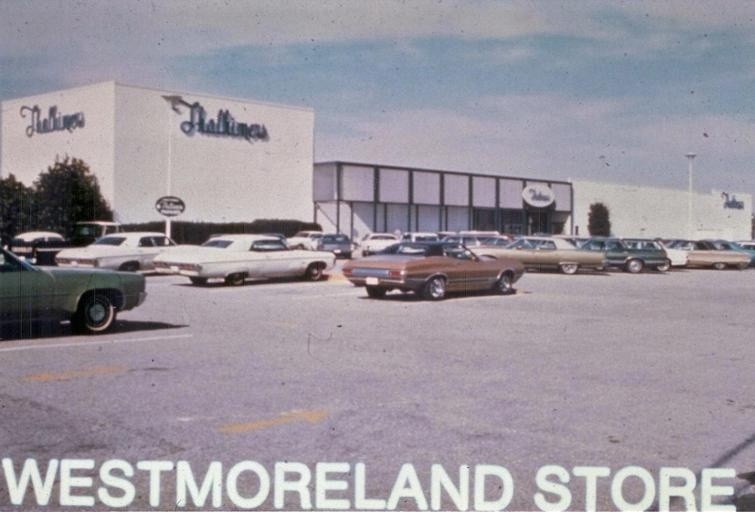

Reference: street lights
[685,150,699,243]
[162,90,182,245]
